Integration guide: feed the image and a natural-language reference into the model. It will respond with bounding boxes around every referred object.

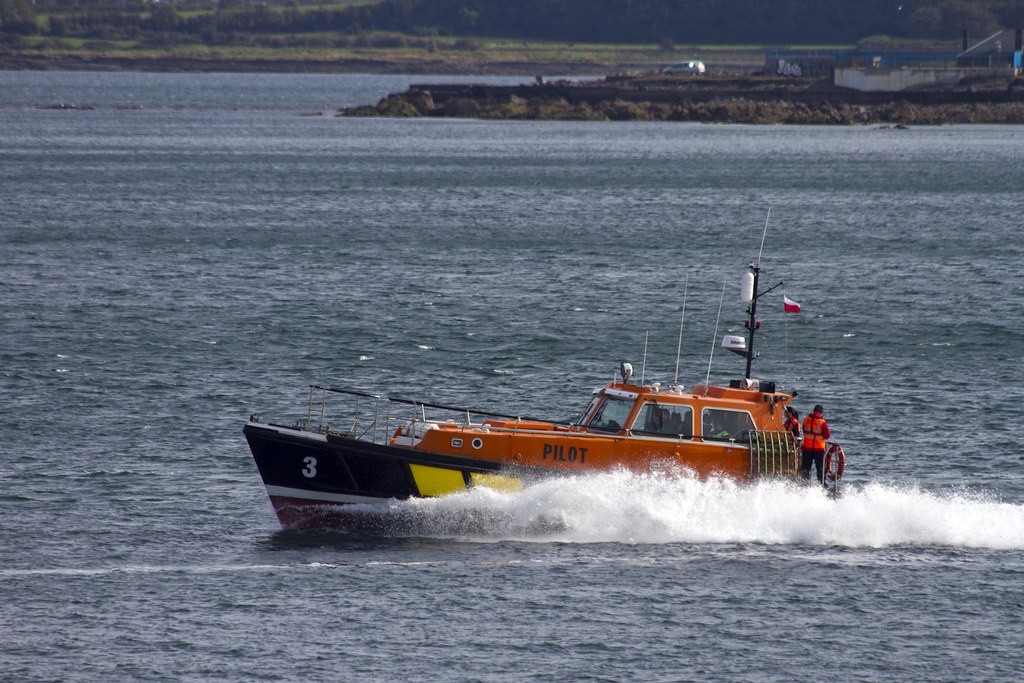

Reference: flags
[783,295,801,312]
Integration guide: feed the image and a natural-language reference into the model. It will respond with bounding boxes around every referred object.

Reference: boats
[241,201,846,541]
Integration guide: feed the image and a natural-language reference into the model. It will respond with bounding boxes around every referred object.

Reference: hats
[814,405,824,414]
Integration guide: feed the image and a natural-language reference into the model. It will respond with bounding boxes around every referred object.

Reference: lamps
[620,362,633,384]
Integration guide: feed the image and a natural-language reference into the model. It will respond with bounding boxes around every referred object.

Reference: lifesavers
[826,445,845,480]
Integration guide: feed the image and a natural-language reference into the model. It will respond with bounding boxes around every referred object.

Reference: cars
[662,61,705,76]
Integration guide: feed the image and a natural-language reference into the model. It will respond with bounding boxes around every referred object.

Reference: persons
[707,420,731,438]
[647,409,677,435]
[802,404,830,488]
[783,407,801,445]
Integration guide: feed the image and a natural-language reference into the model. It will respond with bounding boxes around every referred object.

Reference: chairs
[703,414,712,437]
[671,412,684,434]
[684,411,691,435]
